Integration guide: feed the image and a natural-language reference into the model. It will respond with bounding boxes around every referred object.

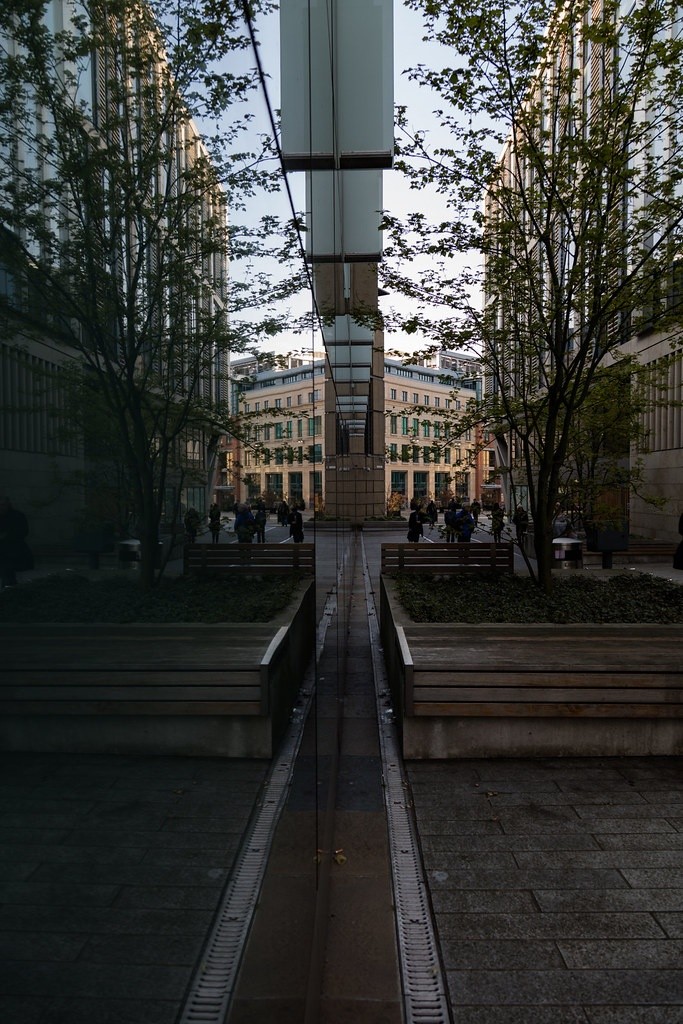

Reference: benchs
[28,537,142,569]
[381,543,515,576]
[583,539,680,560]
[0,623,290,761]
[182,544,316,580]
[394,625,683,756]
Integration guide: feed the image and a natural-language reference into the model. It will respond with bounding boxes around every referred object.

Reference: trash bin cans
[551,538,583,571]
[121,540,141,572]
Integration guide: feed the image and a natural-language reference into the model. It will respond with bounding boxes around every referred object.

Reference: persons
[183,497,304,560]
[406,497,528,565]
[552,500,577,539]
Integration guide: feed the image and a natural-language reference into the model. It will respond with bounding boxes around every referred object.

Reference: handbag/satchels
[407,530,416,542]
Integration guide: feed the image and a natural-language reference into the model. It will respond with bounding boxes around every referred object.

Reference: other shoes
[432,524,435,526]
[495,540,497,543]
[429,524,431,526]
[498,541,501,543]
